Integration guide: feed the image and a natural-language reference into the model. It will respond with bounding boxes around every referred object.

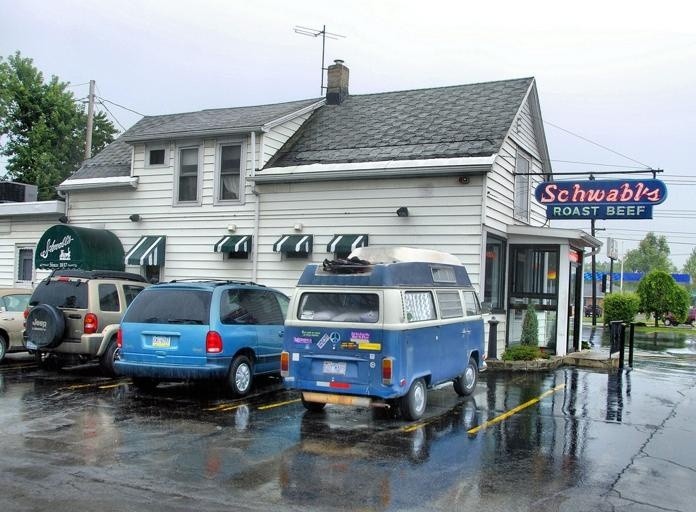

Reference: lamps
[59,216,69,223]
[397,207,408,217]
[129,214,142,222]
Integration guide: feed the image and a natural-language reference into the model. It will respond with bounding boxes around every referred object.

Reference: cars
[662,304,696,327]
[653,311,662,320]
[113,279,292,398]
[0,287,35,363]
[584,304,603,318]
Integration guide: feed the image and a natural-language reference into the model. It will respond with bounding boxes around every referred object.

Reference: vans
[278,244,488,420]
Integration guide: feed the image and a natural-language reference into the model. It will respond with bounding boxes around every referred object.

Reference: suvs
[21,267,158,377]
